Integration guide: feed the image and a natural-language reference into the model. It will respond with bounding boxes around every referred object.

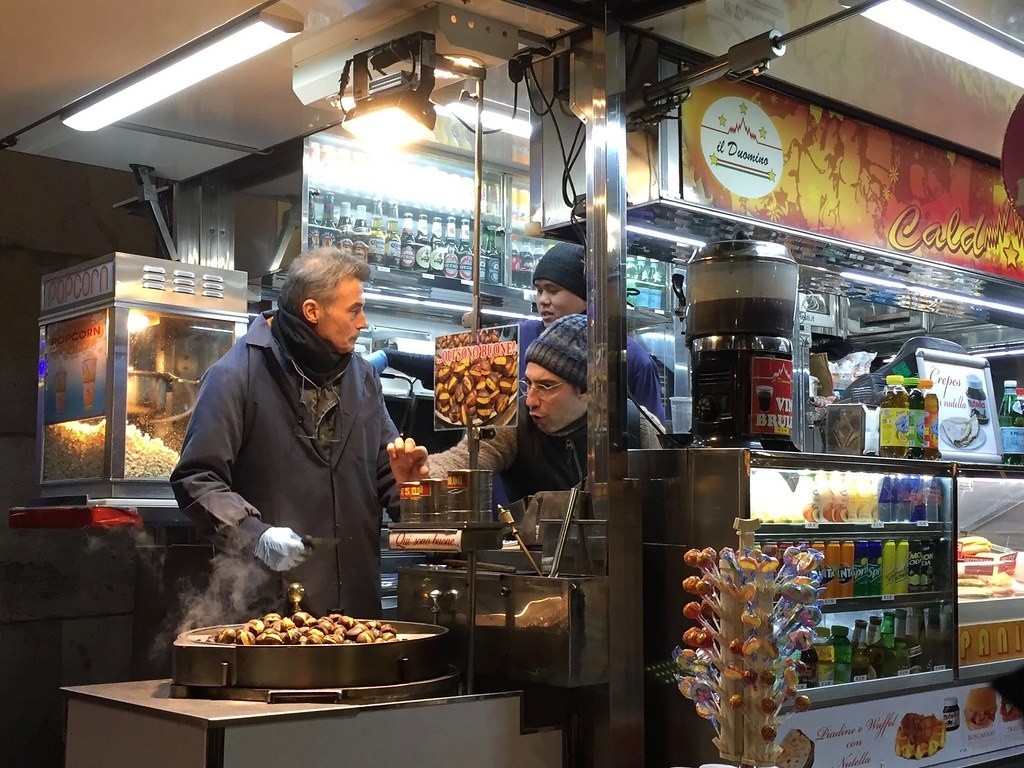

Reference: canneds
[876,473,939,522]
[754,539,950,598]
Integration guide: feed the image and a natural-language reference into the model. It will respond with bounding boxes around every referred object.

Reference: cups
[419,478,448,521]
[399,482,419,521]
[447,470,492,524]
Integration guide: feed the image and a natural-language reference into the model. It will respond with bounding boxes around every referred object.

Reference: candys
[674,545,823,741]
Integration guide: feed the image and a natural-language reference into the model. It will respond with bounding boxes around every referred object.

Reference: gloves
[364,349,388,373]
[253,526,314,572]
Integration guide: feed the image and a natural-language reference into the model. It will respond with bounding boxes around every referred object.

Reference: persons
[463,243,664,521]
[170,247,401,628]
[387,314,665,494]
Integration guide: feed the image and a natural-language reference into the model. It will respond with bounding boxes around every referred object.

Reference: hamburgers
[964,687,997,729]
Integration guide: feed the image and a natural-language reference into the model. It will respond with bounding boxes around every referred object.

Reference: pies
[941,414,980,447]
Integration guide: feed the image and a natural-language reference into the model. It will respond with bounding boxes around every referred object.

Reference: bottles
[648,259,662,311]
[1015,388,1024,413]
[510,234,519,288]
[517,237,533,289]
[636,255,650,309]
[308,189,502,285]
[626,255,639,310]
[750,469,946,523]
[965,374,990,425]
[797,605,948,690]
[878,375,910,459]
[998,380,1024,465]
[942,696,960,731]
[902,377,925,458]
[918,380,939,460]
[532,240,545,290]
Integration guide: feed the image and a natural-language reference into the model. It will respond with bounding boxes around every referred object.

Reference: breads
[895,713,947,760]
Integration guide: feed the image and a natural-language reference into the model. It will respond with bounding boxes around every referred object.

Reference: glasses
[518,378,571,396]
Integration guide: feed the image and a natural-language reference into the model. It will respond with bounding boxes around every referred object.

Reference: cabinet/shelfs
[627,448,958,766]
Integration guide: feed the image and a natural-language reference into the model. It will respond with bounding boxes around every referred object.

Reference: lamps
[59,12,305,132]
[838,1,1024,88]
[339,31,437,148]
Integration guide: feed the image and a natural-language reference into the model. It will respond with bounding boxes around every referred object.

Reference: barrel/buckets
[685,239,800,452]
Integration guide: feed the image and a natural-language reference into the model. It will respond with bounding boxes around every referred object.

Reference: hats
[524,313,587,391]
[533,242,587,302]
